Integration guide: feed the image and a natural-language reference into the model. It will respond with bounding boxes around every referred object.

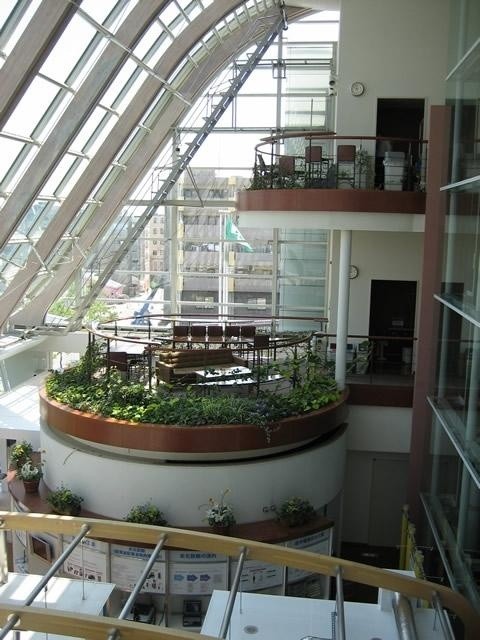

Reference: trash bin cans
[383,151,406,191]
[327,342,356,364]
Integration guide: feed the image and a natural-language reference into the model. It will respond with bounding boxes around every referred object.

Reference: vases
[213,528,235,536]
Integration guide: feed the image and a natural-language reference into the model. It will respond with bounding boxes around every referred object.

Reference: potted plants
[8,439,31,472]
[53,484,84,520]
[20,458,45,495]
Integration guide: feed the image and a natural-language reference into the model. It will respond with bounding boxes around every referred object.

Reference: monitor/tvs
[28,533,51,564]
[183,600,201,615]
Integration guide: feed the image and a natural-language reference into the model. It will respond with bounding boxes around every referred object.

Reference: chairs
[95,314,300,395]
[252,136,369,189]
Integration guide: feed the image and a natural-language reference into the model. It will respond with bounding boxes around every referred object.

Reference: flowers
[202,486,238,526]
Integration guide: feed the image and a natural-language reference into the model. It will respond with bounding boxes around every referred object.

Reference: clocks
[348,264,359,279]
[350,81,364,97]
